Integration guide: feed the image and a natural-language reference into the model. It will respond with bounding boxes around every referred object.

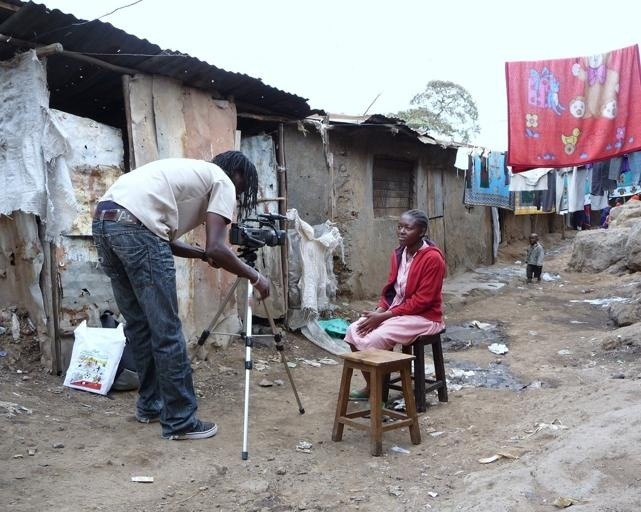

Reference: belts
[95,209,119,221]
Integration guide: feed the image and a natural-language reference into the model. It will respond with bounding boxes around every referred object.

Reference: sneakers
[161,419,218,440]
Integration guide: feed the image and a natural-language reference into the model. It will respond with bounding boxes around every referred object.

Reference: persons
[343,210,446,418]
[576,204,591,231]
[603,215,609,226]
[615,198,622,206]
[525,232,545,283]
[91,149,272,440]
[600,204,612,225]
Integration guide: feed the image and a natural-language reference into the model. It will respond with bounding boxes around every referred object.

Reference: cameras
[229,213,287,247]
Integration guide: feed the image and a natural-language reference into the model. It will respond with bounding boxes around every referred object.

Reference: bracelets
[251,271,260,287]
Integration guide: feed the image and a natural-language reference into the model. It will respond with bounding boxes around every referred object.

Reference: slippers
[349,388,370,401]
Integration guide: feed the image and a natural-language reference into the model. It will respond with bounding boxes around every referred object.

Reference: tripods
[189,248,306,459]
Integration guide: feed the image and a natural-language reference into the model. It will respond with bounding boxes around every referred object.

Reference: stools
[332,348,422,456]
[381,333,448,413]
